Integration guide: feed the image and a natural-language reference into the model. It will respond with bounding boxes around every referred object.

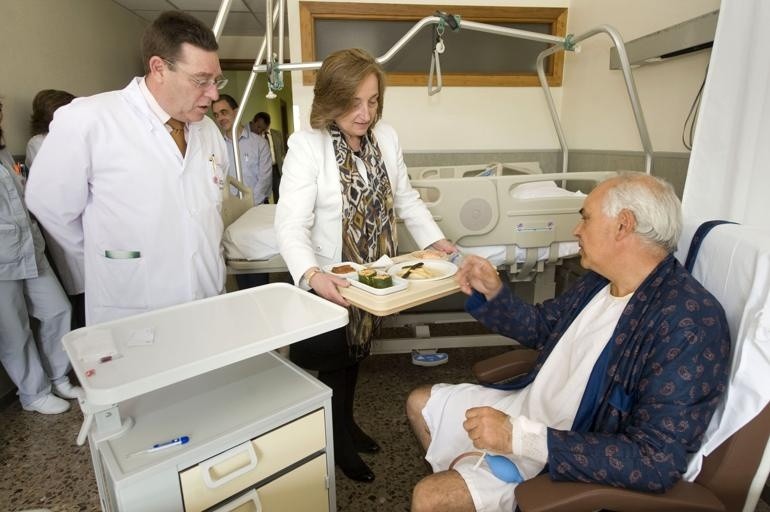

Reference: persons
[22,90,85,298]
[402,169,735,510]
[22,7,231,331]
[211,93,274,205]
[243,111,272,137]
[272,45,460,488]
[1,94,85,417]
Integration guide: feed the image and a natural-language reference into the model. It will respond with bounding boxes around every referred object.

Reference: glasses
[163,58,228,90]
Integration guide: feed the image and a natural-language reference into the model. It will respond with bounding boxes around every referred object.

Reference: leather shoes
[335,448,377,482]
[350,420,382,454]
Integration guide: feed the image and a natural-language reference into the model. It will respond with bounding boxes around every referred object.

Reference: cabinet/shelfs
[77,352,337,512]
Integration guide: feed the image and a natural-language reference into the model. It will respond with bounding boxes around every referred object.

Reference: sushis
[358,269,377,287]
[372,275,392,288]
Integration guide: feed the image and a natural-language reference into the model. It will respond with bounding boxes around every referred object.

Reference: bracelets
[303,266,324,290]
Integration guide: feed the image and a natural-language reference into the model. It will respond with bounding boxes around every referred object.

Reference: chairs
[471,214,770,512]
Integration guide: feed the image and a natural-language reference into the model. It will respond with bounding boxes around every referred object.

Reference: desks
[60,282,349,446]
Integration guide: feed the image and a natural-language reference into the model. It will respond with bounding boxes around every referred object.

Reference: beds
[218,162,595,365]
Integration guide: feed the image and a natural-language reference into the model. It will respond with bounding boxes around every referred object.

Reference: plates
[387,257,460,284]
[322,261,367,280]
[347,269,410,296]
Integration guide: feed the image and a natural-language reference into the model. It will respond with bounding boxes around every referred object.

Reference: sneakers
[52,380,84,399]
[23,392,71,415]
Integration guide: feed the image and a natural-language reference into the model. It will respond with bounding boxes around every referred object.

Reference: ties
[167,117,187,160]
